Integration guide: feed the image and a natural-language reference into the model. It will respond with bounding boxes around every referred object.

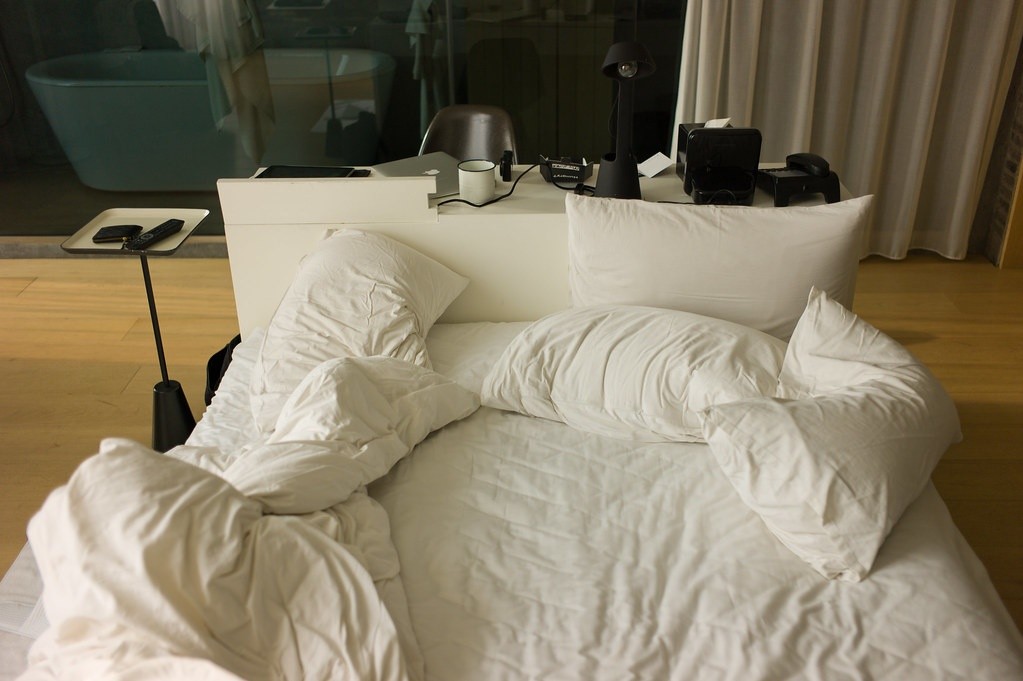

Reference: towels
[153,0,277,163]
[404,1,469,141]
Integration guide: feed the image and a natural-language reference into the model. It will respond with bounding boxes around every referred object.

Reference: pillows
[562,191,874,345]
[476,305,789,443]
[695,284,963,583]
[251,227,471,435]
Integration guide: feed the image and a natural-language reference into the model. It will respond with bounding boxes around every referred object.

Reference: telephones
[757,152,840,207]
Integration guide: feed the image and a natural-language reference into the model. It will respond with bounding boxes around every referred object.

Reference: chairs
[420,103,520,165]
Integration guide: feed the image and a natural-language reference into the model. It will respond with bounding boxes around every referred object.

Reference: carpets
[0,541,50,681]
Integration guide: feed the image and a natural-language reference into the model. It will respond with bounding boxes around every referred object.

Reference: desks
[253,163,856,209]
[61,208,211,452]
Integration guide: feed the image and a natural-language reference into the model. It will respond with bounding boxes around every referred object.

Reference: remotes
[126,218,185,251]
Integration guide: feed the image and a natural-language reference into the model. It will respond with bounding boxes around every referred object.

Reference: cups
[457,158,496,205]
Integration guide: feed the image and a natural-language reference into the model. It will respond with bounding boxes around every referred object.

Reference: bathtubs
[23,48,398,193]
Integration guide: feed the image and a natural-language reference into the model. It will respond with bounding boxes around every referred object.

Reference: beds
[24,180,1023,681]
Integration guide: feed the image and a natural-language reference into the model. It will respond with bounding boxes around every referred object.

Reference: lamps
[594,42,656,200]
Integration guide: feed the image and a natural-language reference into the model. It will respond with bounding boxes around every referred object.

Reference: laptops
[370,150,462,199]
